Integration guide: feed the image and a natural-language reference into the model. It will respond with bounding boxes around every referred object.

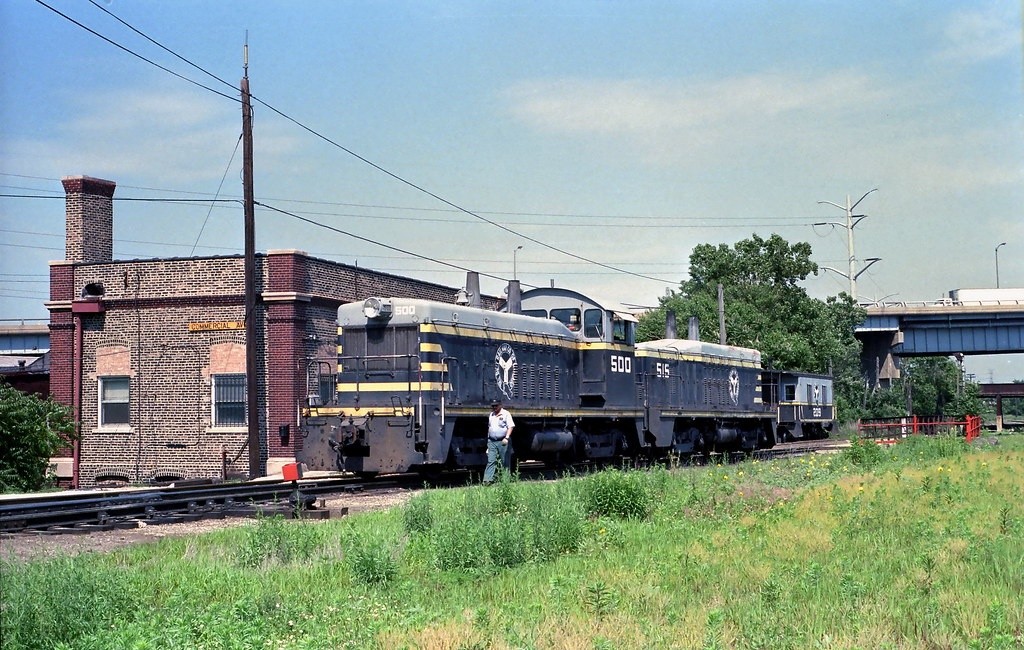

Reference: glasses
[492,405,499,407]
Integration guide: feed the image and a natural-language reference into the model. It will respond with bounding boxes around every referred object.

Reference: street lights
[995,242,1007,288]
[513,245,523,281]
[816,187,881,306]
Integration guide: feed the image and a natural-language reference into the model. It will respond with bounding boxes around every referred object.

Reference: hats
[491,397,501,406]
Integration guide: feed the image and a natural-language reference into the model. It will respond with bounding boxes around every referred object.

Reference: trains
[297,272,778,482]
[761,370,836,439]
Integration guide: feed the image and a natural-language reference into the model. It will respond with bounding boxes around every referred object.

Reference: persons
[482,399,516,487]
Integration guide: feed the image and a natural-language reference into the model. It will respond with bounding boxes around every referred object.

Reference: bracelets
[505,437,509,440]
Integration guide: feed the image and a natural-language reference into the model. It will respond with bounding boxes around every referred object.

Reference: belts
[489,438,503,441]
